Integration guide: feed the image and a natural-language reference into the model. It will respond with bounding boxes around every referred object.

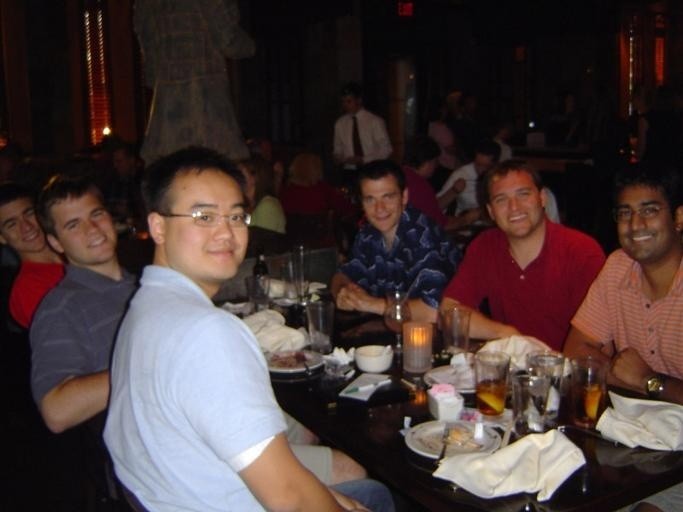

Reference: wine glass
[382,291,412,355]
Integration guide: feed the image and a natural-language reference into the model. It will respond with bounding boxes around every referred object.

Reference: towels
[595,390,683,452]
[473,334,579,377]
[431,428,587,503]
[240,309,305,353]
[258,275,328,299]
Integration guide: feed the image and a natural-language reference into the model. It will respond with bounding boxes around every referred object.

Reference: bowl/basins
[355,345,394,372]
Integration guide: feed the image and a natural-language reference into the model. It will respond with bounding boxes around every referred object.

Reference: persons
[330,157,460,326]
[99,83,581,230]
[440,159,605,356]
[1,188,63,330]
[29,173,366,484]
[101,143,396,512]
[563,163,683,404]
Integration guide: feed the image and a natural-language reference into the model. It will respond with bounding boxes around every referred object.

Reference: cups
[569,356,609,429]
[439,308,472,357]
[245,276,269,303]
[509,368,552,439]
[525,349,565,422]
[472,350,511,420]
[289,246,313,307]
[305,302,336,354]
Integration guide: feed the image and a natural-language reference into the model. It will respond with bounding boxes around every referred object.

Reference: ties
[351,116,364,169]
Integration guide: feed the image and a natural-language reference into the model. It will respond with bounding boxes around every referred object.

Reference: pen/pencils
[569,424,618,446]
[345,379,393,394]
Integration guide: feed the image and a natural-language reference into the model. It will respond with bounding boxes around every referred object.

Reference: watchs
[648,375,664,400]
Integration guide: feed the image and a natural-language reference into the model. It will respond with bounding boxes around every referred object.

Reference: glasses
[158,211,252,228]
[618,204,670,221]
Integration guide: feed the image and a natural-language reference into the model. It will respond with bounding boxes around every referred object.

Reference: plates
[404,420,502,462]
[423,365,475,394]
[264,349,322,374]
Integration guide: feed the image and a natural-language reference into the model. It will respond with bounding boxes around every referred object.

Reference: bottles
[253,247,268,275]
